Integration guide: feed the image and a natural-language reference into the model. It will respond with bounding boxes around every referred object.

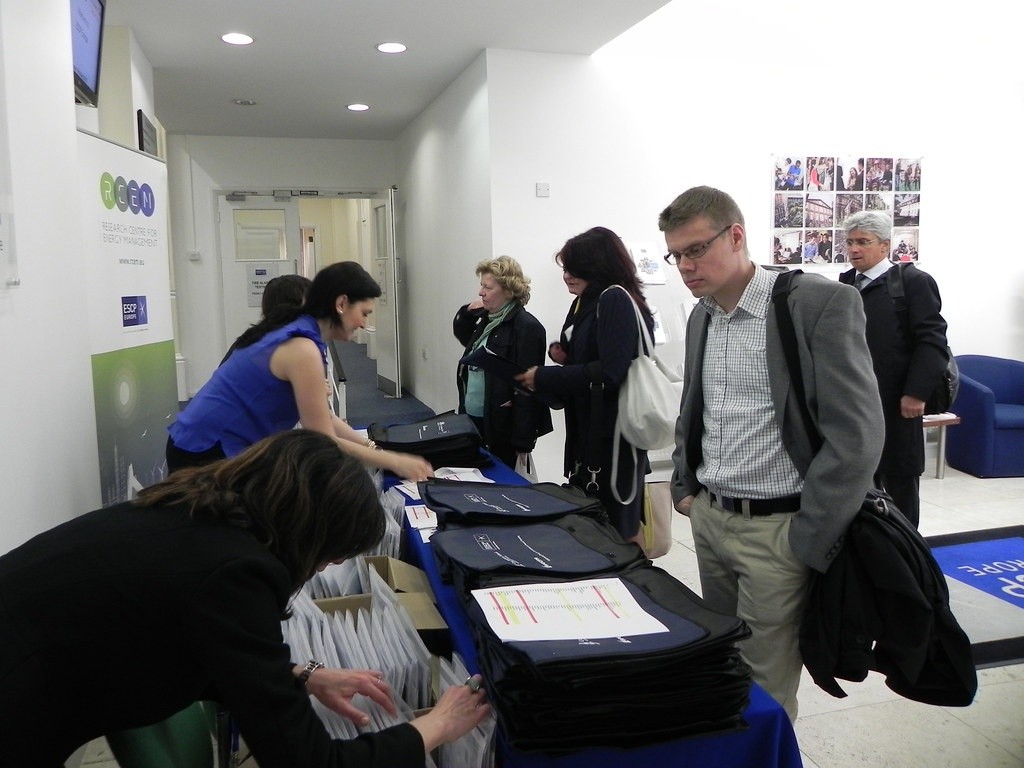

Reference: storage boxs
[310,553,450,767]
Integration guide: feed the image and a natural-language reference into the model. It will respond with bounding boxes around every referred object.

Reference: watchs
[297,659,325,695]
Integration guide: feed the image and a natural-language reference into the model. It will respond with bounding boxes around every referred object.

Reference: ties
[853,274,866,291]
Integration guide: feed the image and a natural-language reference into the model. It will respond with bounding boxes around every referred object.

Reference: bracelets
[364,438,384,452]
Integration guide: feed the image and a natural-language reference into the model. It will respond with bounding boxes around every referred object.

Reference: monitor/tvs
[69,0,106,106]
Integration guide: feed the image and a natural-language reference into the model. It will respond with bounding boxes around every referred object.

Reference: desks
[923,411,962,478]
[352,423,804,767]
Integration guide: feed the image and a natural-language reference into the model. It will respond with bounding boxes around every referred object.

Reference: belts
[704,487,800,516]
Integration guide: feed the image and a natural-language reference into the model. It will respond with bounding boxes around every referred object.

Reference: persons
[453,256,546,482]
[217,275,316,380]
[516,227,655,540]
[165,260,434,484]
[0,429,493,768]
[661,186,886,738]
[773,157,919,266]
[837,211,952,531]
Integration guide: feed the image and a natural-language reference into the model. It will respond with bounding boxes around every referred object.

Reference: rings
[376,676,381,687]
[464,677,481,693]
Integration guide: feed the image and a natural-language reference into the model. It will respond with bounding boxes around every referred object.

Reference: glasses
[842,237,879,249]
[663,225,731,266]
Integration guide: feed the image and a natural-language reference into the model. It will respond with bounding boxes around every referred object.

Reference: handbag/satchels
[932,346,959,414]
[629,480,673,558]
[618,355,685,448]
[514,400,538,453]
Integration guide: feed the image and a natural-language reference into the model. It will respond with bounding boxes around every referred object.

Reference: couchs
[952,352,1023,479]
